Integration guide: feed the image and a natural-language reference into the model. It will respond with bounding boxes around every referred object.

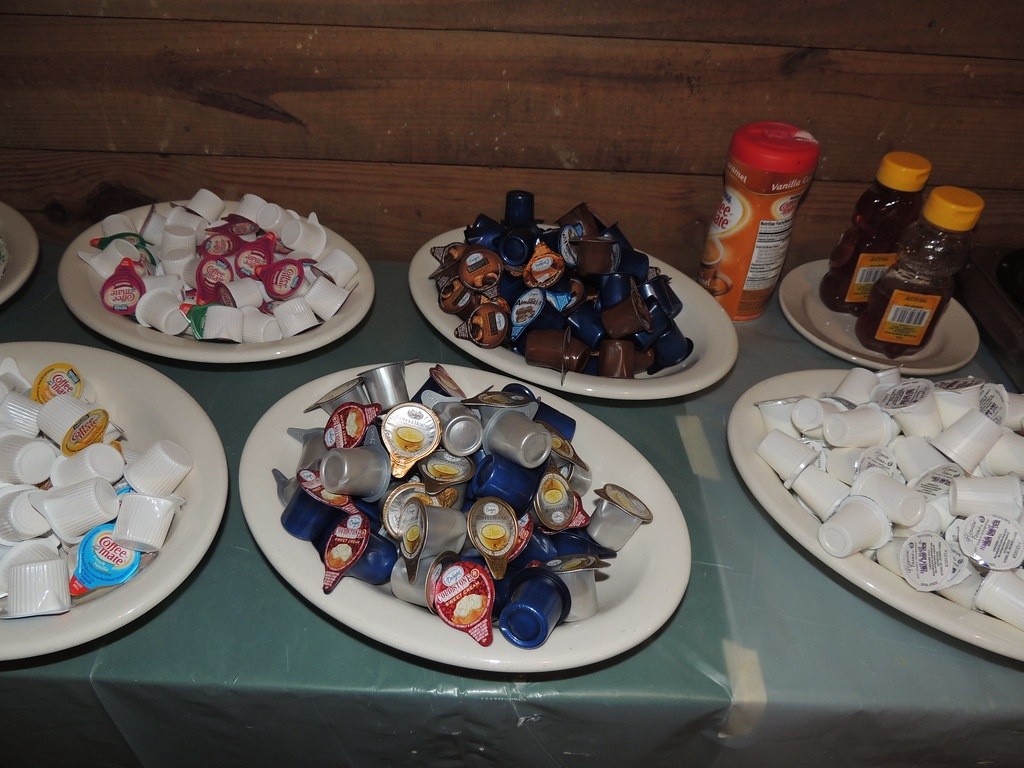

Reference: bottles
[695,123,818,322]
[854,188,987,354]
[820,150,932,314]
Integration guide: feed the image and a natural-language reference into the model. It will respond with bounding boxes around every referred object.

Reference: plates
[58,200,375,362]
[239,361,691,675]
[729,371,1024,661]
[0,341,229,665]
[778,255,980,375]
[0,201,38,305]
[407,224,738,399]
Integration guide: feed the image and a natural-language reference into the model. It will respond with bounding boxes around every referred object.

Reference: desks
[0,242,1023,768]
